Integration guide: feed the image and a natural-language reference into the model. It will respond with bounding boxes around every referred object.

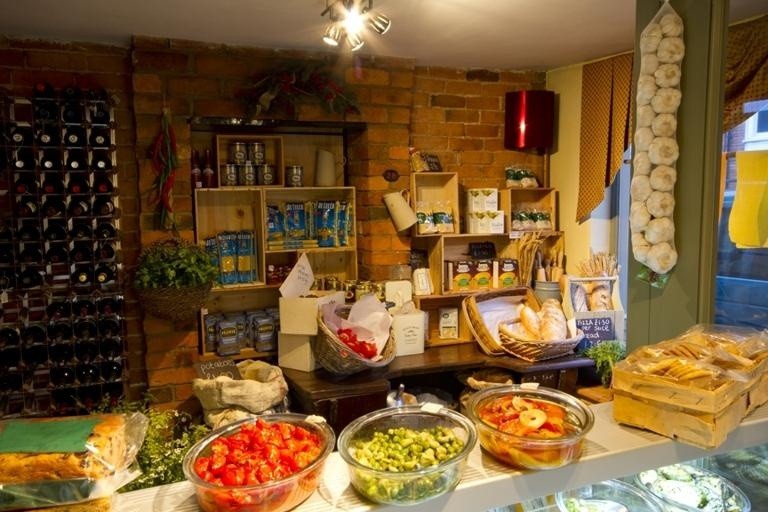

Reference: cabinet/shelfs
[410,172,565,348]
[0,96,131,417]
[192,117,359,360]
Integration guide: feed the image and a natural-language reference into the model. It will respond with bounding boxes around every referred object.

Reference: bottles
[535,248,564,281]
[201,148,213,186]
[190,148,202,188]
[0,79,120,415]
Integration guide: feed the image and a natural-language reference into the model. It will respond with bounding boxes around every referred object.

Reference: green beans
[354,425,467,505]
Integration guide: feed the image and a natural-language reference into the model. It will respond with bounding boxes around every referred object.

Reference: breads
[520,298,568,341]
[0,414,126,487]
[24,497,111,512]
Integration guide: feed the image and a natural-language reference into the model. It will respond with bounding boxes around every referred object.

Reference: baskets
[465,285,585,363]
[311,302,397,378]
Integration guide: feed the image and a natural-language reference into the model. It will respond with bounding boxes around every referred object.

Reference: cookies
[624,331,767,392]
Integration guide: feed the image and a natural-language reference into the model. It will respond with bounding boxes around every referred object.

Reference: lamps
[319,0,391,52]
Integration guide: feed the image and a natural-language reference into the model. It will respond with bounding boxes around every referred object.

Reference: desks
[281,339,593,452]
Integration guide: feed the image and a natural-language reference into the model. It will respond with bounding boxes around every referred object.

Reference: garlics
[628,11,687,276]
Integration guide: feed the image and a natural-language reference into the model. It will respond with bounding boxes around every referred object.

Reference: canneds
[285,164,303,188]
[310,275,391,305]
[219,141,276,187]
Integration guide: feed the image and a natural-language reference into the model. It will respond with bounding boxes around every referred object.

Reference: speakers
[503,89,557,154]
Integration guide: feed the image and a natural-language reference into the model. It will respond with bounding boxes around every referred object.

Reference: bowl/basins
[182,414,335,512]
[633,465,752,512]
[335,405,476,508]
[553,478,661,512]
[467,386,593,472]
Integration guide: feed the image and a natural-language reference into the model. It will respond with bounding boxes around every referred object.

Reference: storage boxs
[277,291,346,372]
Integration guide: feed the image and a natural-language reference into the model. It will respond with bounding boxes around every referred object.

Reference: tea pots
[380,188,419,234]
[311,146,348,187]
